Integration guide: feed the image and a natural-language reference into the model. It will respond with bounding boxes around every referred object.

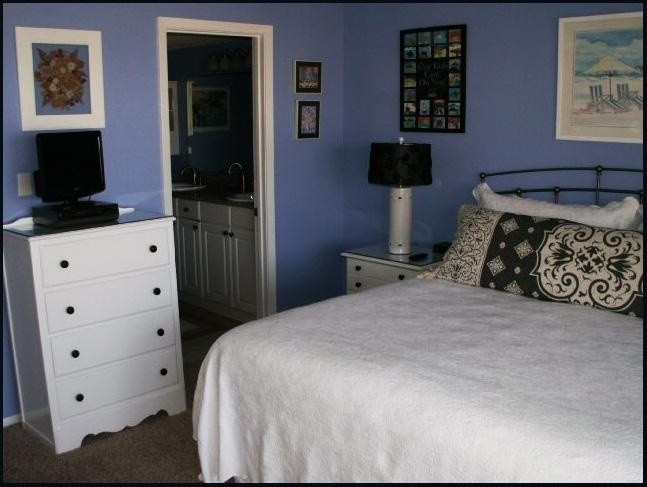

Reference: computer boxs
[33,202,118,228]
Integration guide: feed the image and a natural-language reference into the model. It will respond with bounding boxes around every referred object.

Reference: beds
[191,165,645,483]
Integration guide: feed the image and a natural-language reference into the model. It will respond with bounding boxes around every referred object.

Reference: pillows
[472,182,642,233]
[418,203,642,314]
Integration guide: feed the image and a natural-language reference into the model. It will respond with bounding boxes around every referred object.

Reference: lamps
[368,138,432,254]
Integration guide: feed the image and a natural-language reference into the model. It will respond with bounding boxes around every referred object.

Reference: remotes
[409,253,428,261]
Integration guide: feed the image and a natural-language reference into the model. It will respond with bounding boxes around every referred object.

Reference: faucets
[179,166,197,184]
[227,162,245,191]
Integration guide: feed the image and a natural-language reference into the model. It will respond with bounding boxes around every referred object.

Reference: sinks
[226,193,252,201]
[172,183,206,191]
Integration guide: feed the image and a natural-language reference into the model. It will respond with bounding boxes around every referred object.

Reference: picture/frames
[293,59,323,95]
[294,99,319,138]
[14,27,106,131]
[555,12,643,144]
[400,23,466,134]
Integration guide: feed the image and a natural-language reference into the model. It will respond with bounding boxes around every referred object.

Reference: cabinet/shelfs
[3,207,186,455]
[341,246,443,294]
[173,195,258,323]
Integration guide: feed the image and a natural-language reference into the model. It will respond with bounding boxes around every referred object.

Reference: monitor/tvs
[33,129,106,203]
